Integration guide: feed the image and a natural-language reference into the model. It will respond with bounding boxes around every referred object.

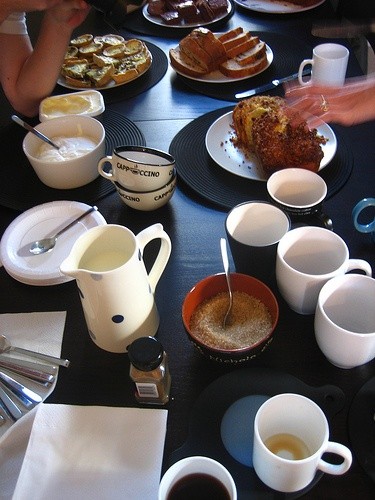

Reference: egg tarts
[63,33,144,59]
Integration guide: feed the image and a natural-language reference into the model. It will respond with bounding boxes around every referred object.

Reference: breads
[168,26,268,78]
[230,96,324,178]
[147,0,228,25]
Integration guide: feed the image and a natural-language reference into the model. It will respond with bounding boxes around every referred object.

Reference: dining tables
[0,0,375,500]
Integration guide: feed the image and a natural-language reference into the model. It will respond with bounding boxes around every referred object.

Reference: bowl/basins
[22,115,105,190]
[180,271,279,366]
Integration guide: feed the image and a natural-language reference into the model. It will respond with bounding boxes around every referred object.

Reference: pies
[60,53,151,88]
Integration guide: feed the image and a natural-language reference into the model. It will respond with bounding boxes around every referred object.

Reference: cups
[98,144,177,210]
[297,43,350,87]
[224,200,292,291]
[313,274,375,370]
[252,392,352,492]
[158,456,238,500]
[350,197,375,234]
[266,168,334,232]
[275,226,373,315]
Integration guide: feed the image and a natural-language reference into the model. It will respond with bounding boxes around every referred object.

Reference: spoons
[220,239,234,329]
[29,206,99,255]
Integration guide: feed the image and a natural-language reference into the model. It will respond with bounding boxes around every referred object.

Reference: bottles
[127,336,172,405]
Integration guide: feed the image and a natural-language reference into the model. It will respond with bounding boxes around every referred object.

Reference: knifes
[234,69,311,100]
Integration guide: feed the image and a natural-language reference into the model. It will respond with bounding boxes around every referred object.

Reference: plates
[57,49,153,90]
[0,201,108,286]
[234,0,326,13]
[142,0,232,28]
[204,104,338,182]
[169,32,273,82]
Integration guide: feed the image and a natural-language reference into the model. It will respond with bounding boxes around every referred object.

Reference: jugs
[58,222,172,354]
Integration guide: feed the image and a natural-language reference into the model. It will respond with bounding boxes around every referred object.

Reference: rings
[321,94,328,112]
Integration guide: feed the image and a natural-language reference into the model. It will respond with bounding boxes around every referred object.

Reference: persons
[284,72,375,132]
[0,0,94,118]
